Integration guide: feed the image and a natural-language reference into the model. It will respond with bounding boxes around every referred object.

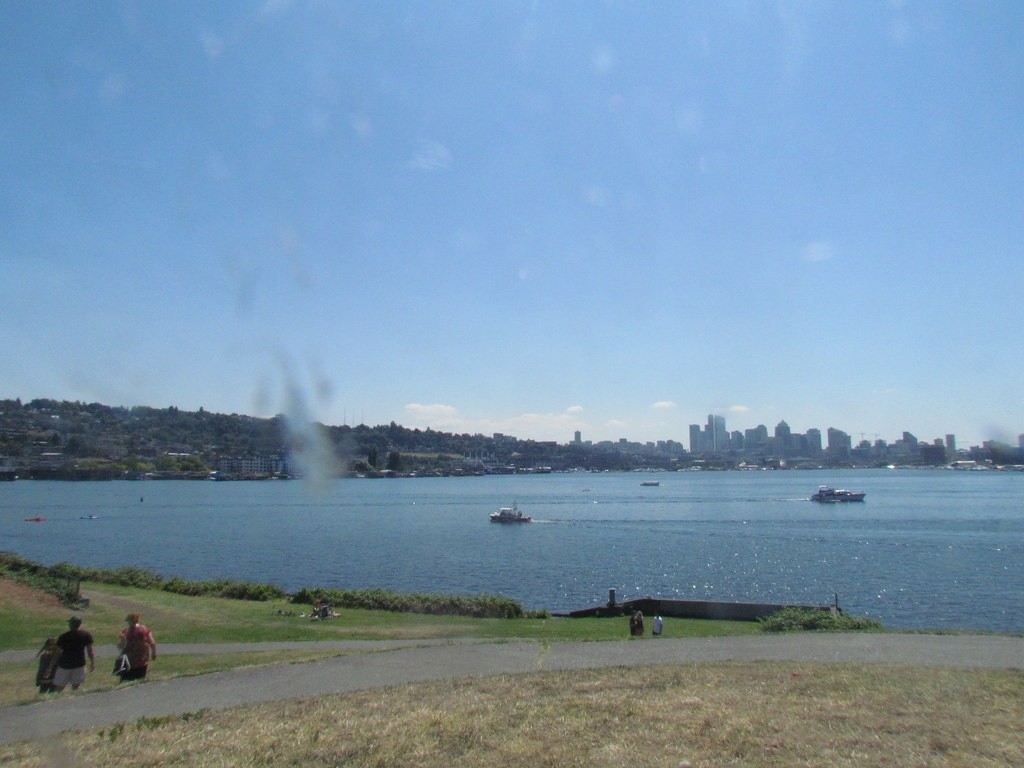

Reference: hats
[67,616,81,622]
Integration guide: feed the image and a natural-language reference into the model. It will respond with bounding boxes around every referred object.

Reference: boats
[640,481,659,486]
[810,485,851,503]
[489,508,532,523]
[846,491,866,502]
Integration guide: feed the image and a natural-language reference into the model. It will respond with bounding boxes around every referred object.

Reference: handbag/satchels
[113,655,131,674]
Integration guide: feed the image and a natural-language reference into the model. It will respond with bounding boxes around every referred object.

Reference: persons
[35,637,63,694]
[634,611,644,636]
[311,599,337,619]
[115,614,158,685]
[651,611,664,635]
[43,616,97,694]
[630,609,639,635]
